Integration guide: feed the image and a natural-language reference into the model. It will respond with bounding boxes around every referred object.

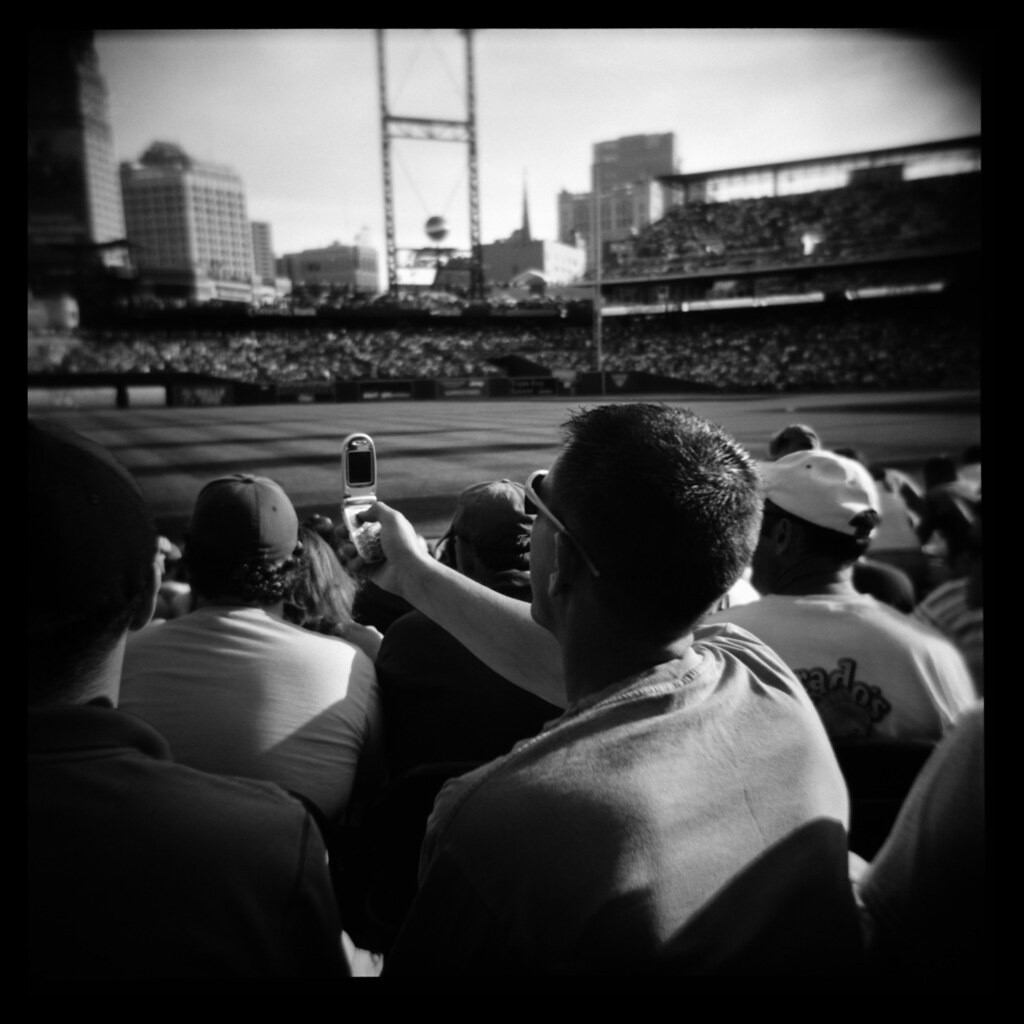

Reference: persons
[28,175,982,389]
[28,407,982,989]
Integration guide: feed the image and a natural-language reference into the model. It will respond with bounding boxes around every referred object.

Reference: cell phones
[340,432,387,564]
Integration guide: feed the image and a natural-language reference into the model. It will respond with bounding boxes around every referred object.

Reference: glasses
[525,465,601,577]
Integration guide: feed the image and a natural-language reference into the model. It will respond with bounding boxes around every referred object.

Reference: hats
[752,448,879,540]
[19,423,160,628]
[190,470,300,560]
[433,477,531,549]
